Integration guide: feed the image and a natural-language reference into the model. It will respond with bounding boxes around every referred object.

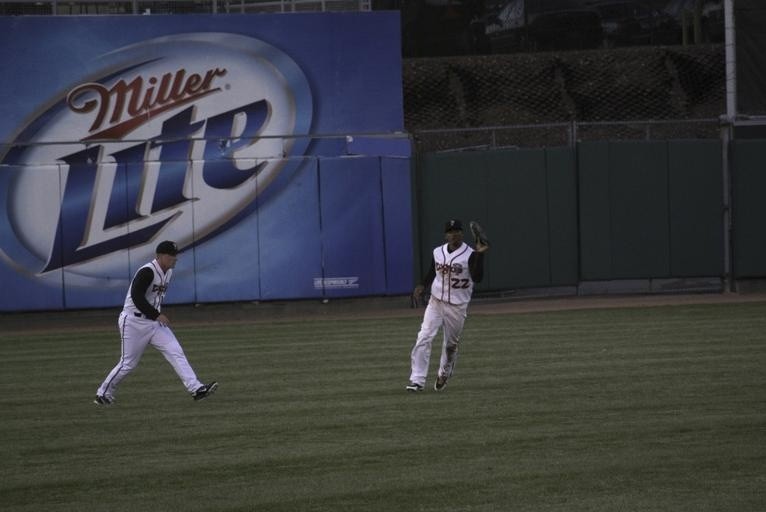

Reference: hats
[156,241,182,254]
[444,220,463,234]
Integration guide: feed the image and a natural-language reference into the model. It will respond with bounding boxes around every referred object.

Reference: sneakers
[433,375,448,392]
[406,383,424,392]
[93,395,116,405]
[191,381,218,402]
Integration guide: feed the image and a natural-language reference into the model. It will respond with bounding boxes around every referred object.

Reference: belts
[134,312,142,317]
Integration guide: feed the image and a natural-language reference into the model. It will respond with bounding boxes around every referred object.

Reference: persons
[92,238,218,407]
[404,218,492,392]
